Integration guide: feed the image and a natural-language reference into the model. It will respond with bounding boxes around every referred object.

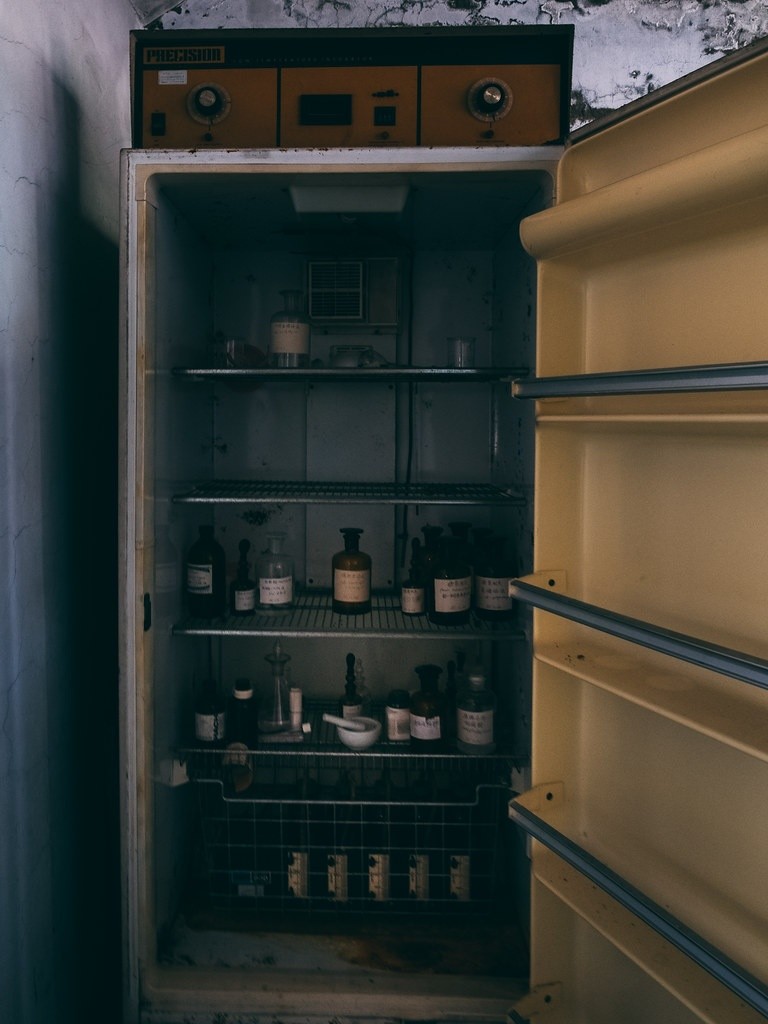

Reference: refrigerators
[117,25,768,1024]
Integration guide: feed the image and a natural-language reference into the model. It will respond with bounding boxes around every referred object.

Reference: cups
[447,337,476,368]
[225,342,245,369]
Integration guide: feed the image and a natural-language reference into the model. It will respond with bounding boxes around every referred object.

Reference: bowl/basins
[337,716,383,750]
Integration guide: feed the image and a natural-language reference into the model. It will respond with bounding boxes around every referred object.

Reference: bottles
[259,652,298,734]
[255,532,295,615]
[267,290,312,369]
